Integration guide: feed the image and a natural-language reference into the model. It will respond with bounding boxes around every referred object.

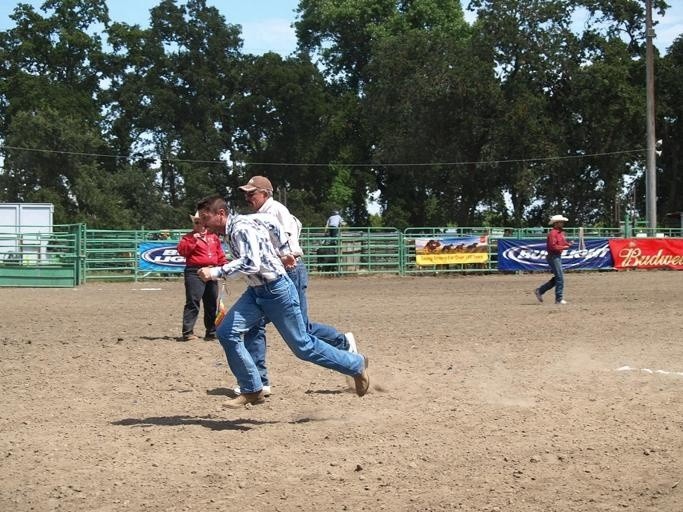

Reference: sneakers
[221,389,266,407]
[555,299,568,305]
[205,333,218,340]
[344,331,357,353]
[183,335,199,341]
[352,353,370,397]
[533,288,544,303]
[233,384,271,397]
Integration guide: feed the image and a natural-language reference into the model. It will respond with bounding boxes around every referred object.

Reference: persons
[177,210,230,342]
[196,193,370,410]
[232,176,358,397]
[534,214,572,305]
[325,209,347,245]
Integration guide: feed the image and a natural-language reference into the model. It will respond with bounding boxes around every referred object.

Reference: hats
[188,210,201,222]
[237,176,274,193]
[548,214,569,226]
